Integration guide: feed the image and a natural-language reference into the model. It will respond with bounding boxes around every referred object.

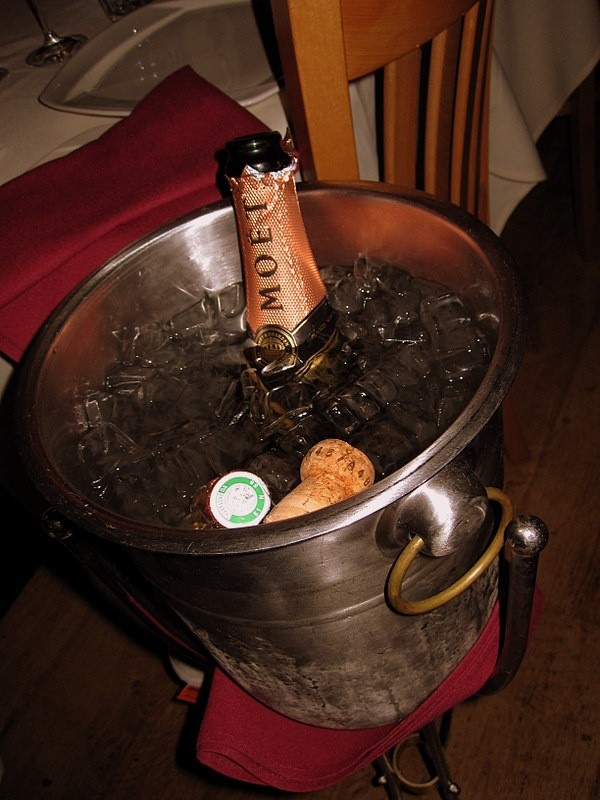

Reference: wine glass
[24,0,87,65]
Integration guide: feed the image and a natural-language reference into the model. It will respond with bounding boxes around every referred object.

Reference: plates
[38,0,280,117]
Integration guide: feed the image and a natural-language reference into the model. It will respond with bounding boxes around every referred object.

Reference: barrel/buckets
[18,180,524,730]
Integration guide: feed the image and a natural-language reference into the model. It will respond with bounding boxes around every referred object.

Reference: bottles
[221,131,345,403]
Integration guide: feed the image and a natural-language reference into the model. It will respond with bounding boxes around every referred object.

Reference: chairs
[264,0,498,233]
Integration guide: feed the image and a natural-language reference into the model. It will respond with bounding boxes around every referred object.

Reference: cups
[97,0,152,23]
